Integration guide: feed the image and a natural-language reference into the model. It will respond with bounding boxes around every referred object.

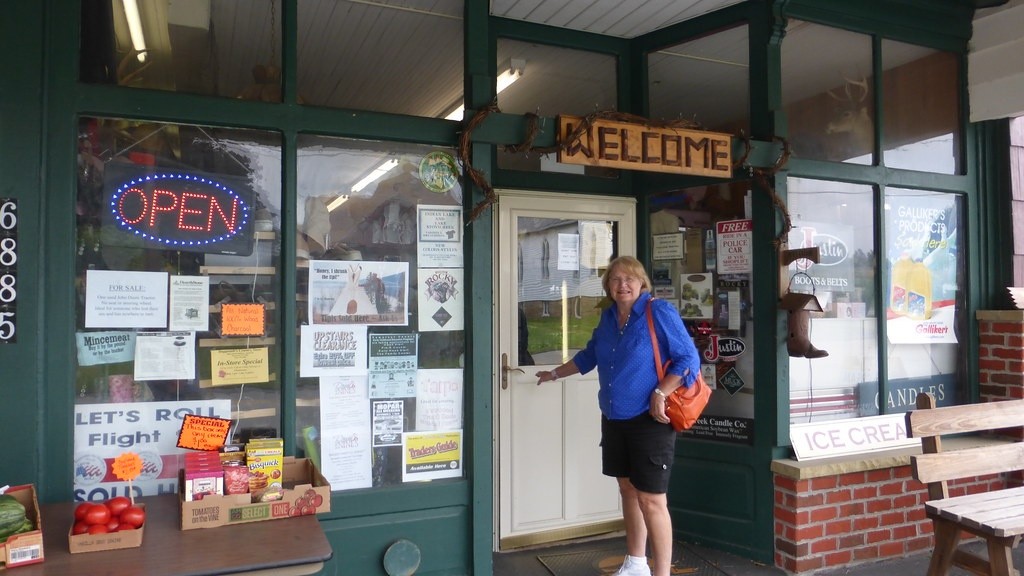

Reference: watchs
[654,388,665,397]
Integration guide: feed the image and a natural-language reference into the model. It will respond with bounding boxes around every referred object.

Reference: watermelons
[0,494,34,543]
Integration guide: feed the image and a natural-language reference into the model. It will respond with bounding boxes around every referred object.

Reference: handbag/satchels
[646,296,713,434]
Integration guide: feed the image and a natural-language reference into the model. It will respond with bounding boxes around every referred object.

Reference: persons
[535,257,701,576]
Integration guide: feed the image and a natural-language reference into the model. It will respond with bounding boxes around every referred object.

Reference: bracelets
[551,367,559,378]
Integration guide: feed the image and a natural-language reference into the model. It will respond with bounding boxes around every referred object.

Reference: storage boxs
[828,303,866,319]
[0,484,45,571]
[68,502,146,554]
[177,455,332,533]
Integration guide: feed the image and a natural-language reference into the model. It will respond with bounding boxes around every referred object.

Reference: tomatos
[72,496,145,535]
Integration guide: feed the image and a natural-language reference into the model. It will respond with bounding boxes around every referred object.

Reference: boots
[787,309,829,359]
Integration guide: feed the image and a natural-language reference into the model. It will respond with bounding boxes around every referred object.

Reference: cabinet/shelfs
[197,231,319,420]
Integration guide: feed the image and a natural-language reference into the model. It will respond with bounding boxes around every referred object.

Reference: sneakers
[254,209,273,232]
[610,553,652,576]
[327,242,363,261]
[295,229,310,260]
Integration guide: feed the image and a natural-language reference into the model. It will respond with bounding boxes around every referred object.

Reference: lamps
[349,154,401,193]
[325,190,350,213]
[437,58,527,121]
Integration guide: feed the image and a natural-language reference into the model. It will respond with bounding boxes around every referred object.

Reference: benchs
[903,392,1024,576]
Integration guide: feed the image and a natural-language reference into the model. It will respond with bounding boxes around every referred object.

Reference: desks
[0,493,332,576]
